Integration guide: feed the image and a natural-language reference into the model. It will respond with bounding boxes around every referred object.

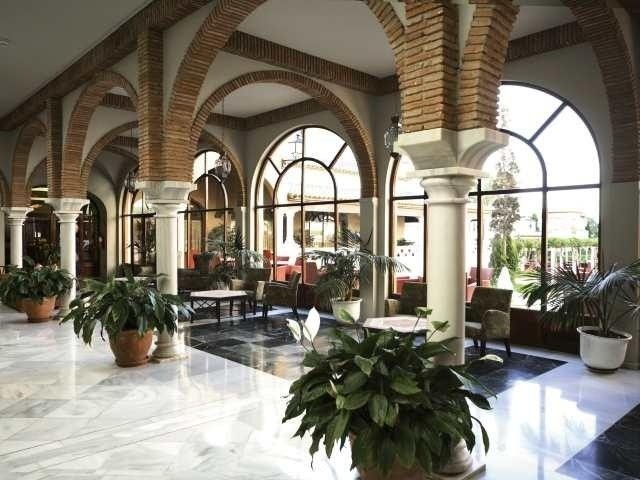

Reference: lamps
[214,98,232,180]
[125,129,137,193]
[383,72,399,159]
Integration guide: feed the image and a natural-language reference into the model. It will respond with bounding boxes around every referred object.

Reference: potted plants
[56,266,196,371]
[1,256,78,323]
[296,228,408,329]
[517,254,639,374]
[278,303,497,480]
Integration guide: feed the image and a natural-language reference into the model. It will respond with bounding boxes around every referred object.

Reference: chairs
[385,281,428,317]
[465,285,515,358]
[177,265,302,324]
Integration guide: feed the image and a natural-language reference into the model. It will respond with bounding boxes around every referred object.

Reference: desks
[360,314,428,342]
